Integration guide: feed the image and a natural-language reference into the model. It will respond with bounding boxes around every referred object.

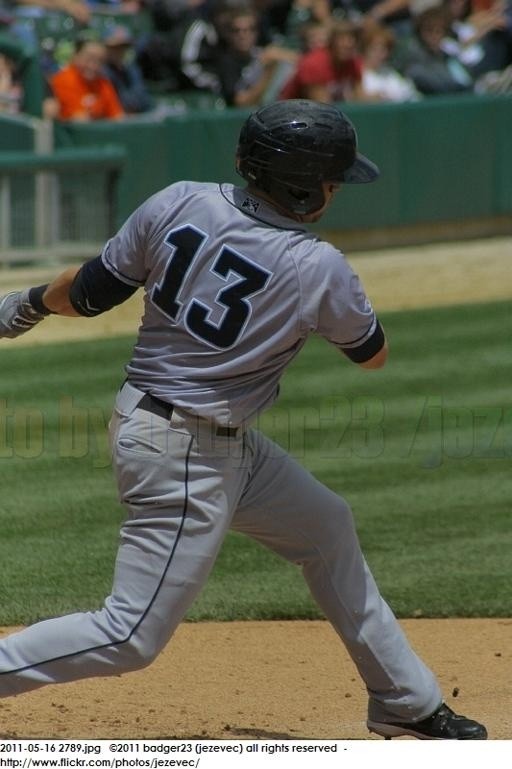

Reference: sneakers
[367,702,488,740]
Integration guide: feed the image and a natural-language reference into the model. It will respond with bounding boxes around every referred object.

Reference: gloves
[1,284,47,338]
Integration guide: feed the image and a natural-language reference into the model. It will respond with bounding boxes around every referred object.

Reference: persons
[0,99,490,738]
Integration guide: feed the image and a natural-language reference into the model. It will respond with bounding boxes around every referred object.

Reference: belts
[135,393,237,437]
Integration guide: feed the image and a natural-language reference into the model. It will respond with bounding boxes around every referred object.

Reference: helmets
[236,99,380,215]
